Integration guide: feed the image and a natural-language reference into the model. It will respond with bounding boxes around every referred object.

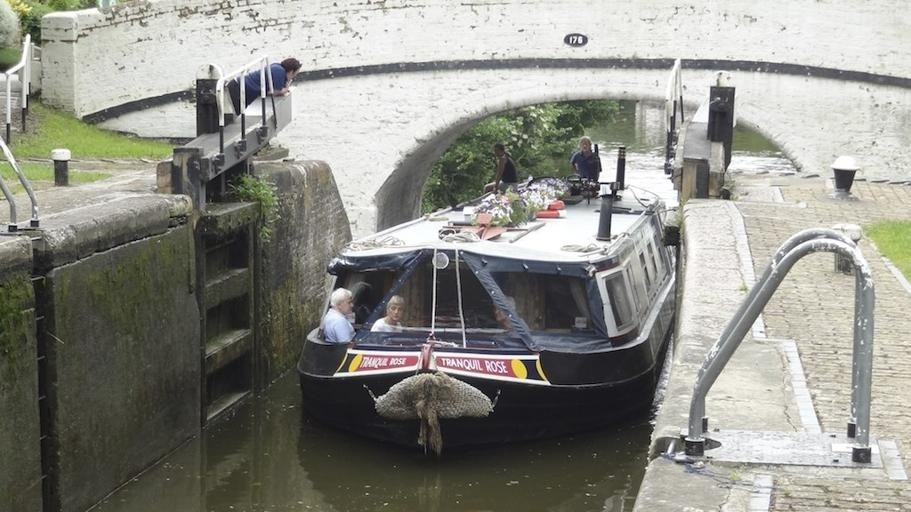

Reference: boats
[291,139,684,458]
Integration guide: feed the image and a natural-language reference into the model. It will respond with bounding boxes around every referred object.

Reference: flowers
[475,178,568,227]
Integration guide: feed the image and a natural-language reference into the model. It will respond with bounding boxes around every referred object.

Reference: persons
[568,137,603,182]
[369,294,409,337]
[228,56,303,117]
[483,143,520,190]
[351,280,376,324]
[320,285,359,344]
[491,294,519,330]
